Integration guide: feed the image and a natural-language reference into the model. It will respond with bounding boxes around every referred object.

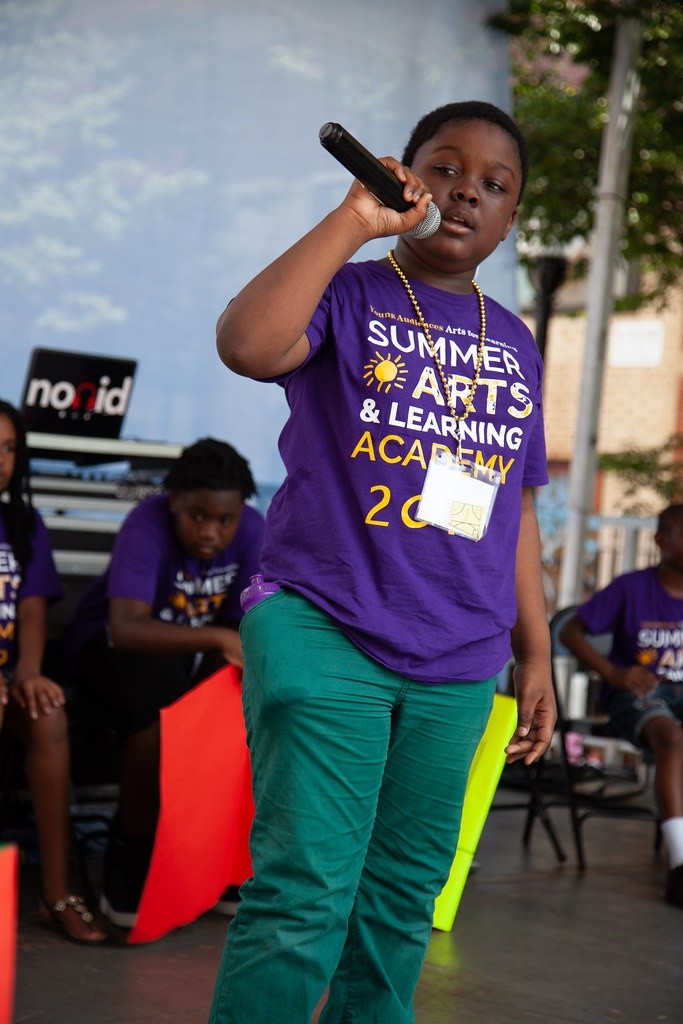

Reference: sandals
[36,896,112,945]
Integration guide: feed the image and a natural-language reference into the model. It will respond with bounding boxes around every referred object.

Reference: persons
[57,436,267,926]
[210,101,558,1024]
[0,399,110,943]
[558,499,683,910]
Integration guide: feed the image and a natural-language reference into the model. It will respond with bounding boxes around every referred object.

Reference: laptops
[19,348,138,439]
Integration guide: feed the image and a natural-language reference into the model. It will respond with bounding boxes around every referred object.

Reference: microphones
[318,123,442,241]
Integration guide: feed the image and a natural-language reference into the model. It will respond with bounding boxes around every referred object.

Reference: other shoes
[665,863,683,909]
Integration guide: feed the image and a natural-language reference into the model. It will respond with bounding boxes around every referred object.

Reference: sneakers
[211,886,242,916]
[99,834,149,928]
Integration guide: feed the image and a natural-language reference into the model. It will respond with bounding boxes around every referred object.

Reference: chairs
[523,607,654,872]
